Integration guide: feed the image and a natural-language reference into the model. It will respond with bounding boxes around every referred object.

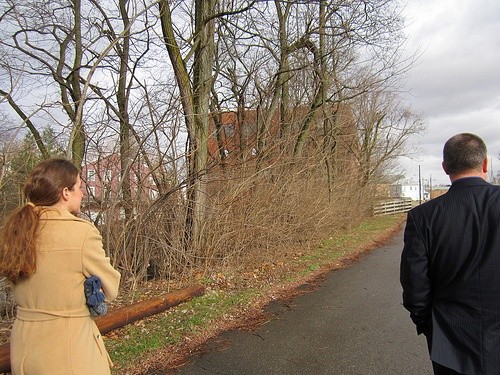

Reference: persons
[0,158,121,375]
[397,130,500,375]
